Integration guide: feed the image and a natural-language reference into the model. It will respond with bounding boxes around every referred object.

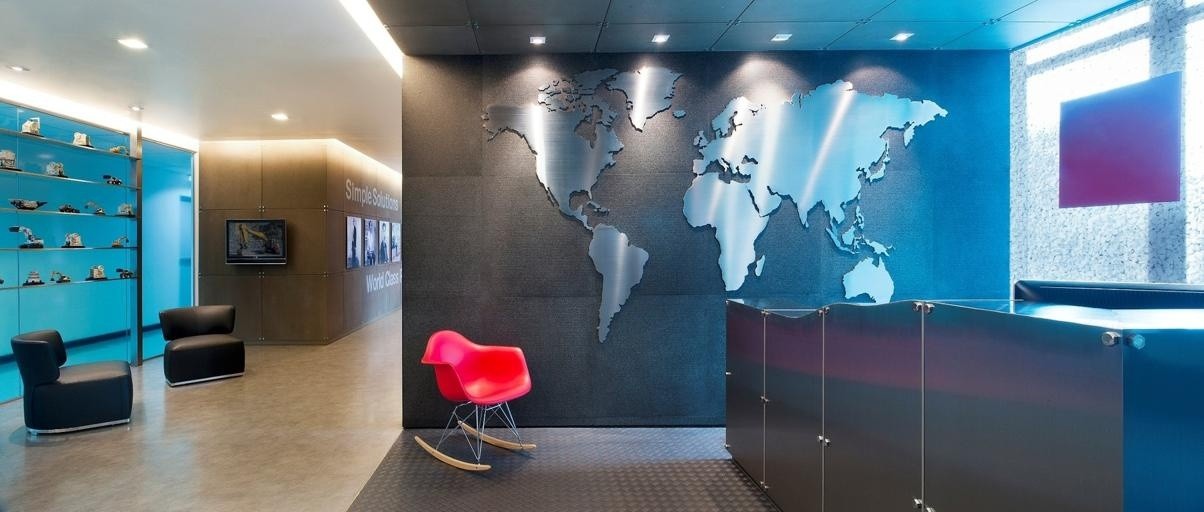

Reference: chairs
[414,329,536,474]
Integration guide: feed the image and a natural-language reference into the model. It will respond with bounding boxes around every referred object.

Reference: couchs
[158,304,246,386]
[10,329,133,435]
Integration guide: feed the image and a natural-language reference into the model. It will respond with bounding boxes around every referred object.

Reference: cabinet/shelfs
[0,94,140,410]
[141,136,199,365]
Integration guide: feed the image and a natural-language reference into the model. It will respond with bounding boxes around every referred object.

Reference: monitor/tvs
[225,218,287,265]
[1014,279,1203,310]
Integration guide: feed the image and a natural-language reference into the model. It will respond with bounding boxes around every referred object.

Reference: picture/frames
[392,221,402,263]
[364,218,377,268]
[379,220,390,265]
[346,216,361,268]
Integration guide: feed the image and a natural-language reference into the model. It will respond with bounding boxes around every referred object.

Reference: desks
[722,293,1174,510]
